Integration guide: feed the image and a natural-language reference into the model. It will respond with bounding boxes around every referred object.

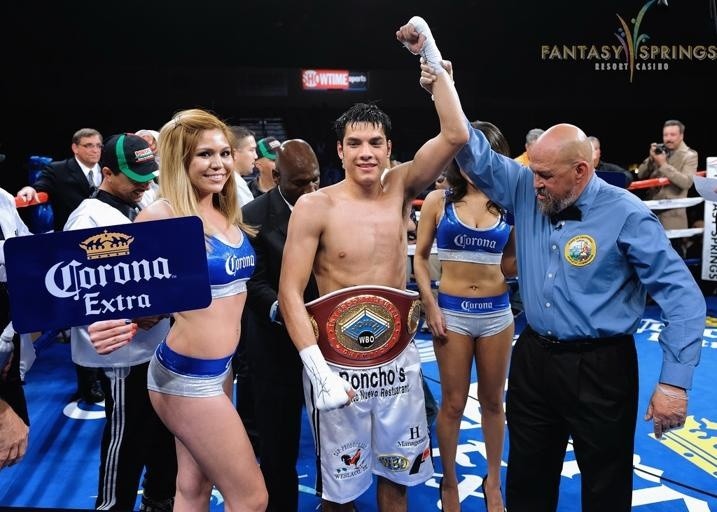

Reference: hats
[98,129,164,181]
[257,137,283,161]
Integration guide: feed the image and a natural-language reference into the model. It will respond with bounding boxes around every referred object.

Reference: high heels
[440,476,461,512]
[482,473,504,511]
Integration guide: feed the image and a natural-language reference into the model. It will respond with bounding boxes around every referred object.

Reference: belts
[524,322,636,353]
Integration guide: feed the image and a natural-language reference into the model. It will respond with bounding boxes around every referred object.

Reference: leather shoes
[79,384,104,407]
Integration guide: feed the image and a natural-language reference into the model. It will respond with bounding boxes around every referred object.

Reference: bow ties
[550,205,584,225]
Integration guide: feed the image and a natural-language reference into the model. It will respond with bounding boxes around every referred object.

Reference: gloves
[644,384,688,440]
[300,344,352,412]
[399,15,446,73]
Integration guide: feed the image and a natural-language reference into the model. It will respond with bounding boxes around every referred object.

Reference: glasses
[78,142,105,148]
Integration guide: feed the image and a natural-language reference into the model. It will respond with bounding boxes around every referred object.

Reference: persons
[584,136,633,194]
[278,16,470,512]
[640,119,698,262]
[66,132,178,511]
[247,137,281,198]
[224,126,258,209]
[511,129,545,164]
[420,56,706,512]
[0,398,30,467]
[91,109,268,511]
[236,138,320,511]
[0,187,34,426]
[415,122,516,511]
[17,129,105,405]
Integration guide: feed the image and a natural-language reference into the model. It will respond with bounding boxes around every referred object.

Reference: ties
[87,170,96,196]
